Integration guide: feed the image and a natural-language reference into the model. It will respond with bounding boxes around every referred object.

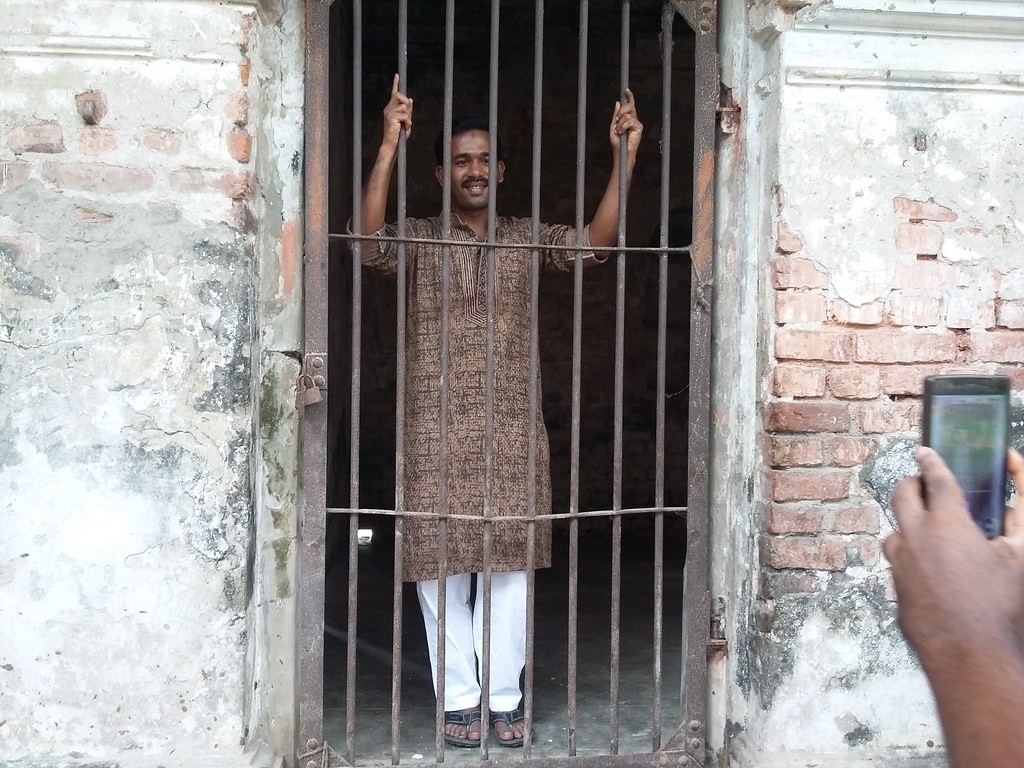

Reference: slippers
[444,712,480,748]
[491,709,535,747]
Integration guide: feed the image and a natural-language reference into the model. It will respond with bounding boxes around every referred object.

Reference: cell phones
[922,375,1010,541]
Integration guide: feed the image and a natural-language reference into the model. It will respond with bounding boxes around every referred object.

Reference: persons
[346,72,644,747]
[942,423,986,518]
[882,445,1024,768]
